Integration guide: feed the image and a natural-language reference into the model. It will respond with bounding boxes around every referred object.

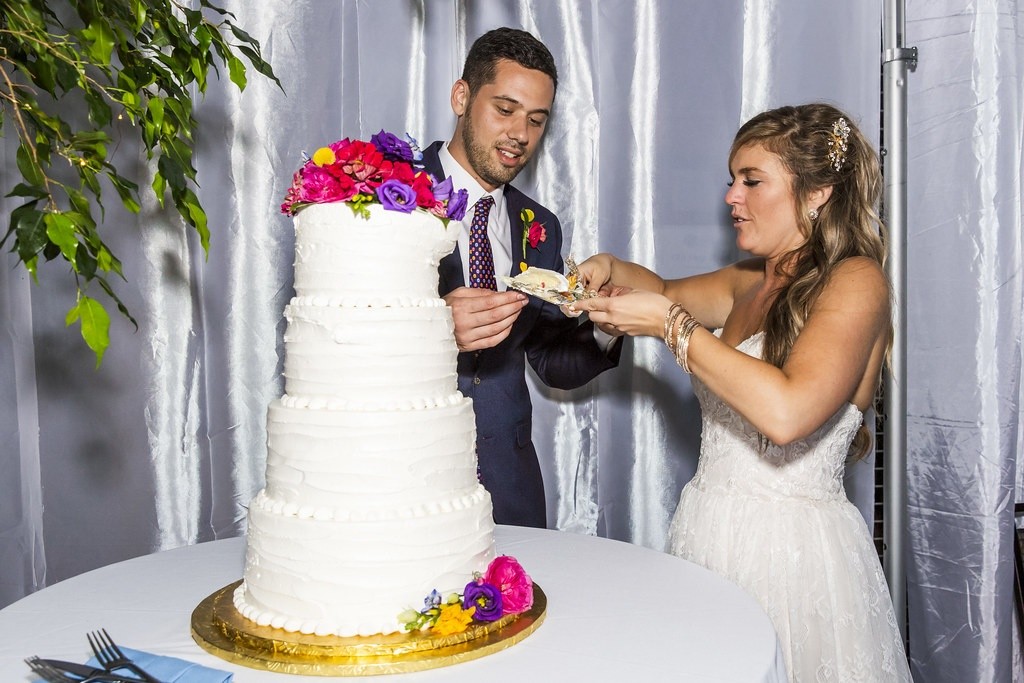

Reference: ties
[468,196,499,293]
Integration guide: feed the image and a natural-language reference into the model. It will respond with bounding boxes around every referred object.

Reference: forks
[85,628,159,682]
[21,654,145,682]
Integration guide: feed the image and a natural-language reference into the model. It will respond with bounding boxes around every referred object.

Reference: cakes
[230,201,496,637]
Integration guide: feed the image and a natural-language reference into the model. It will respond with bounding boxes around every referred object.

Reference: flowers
[401,553,535,637]
[521,207,547,270]
[280,127,472,229]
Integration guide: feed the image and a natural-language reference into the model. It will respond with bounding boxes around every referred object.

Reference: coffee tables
[0,524,788,682]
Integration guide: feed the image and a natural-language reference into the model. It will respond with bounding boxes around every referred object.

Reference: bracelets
[661,301,702,373]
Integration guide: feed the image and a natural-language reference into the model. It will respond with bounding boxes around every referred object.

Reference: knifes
[31,659,147,682]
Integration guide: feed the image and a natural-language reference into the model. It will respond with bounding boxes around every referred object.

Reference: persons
[562,101,917,683]
[404,26,627,529]
